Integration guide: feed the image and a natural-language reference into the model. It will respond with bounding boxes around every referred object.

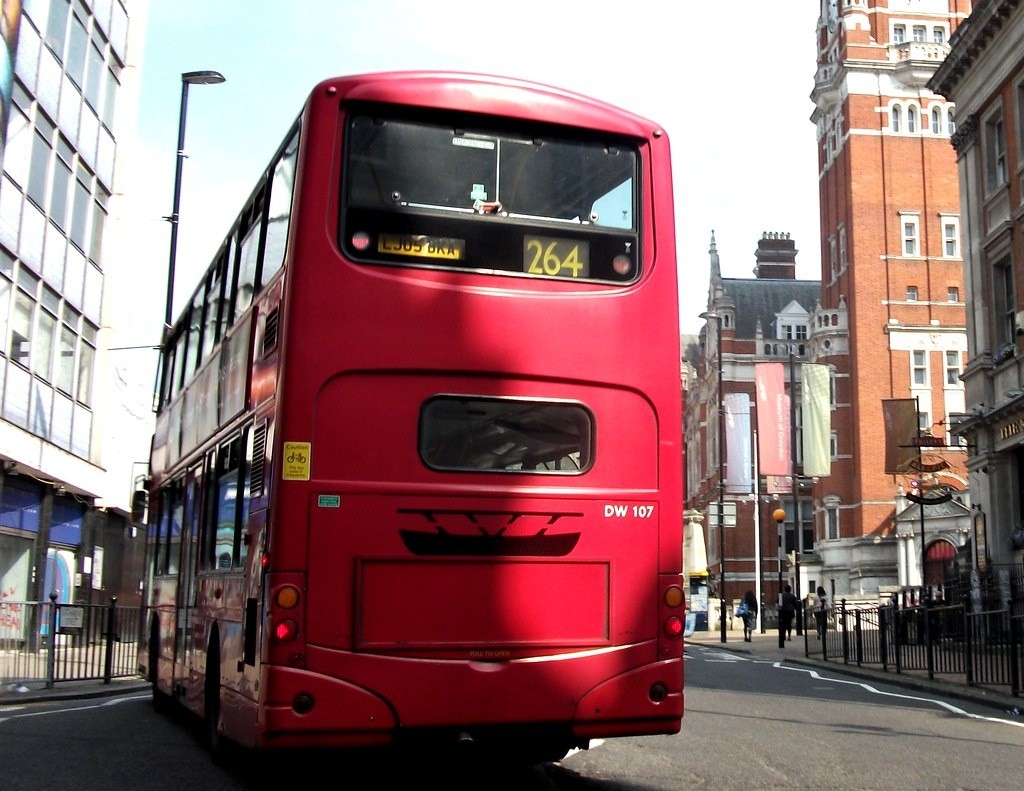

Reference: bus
[127,70,688,772]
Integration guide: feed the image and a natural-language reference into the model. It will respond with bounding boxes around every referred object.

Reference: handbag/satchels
[823,597,833,611]
[735,599,748,618]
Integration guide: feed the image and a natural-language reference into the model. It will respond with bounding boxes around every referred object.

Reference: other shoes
[817,635,820,639]
[784,636,786,640]
[748,638,751,642]
[788,638,791,640]
[744,638,748,642]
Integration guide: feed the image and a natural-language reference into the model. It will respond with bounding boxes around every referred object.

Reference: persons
[814,586,830,640]
[775,585,796,641]
[735,590,758,642]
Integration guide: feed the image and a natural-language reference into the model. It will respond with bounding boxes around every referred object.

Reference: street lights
[771,508,787,610]
[165,68,224,338]
[697,311,727,644]
